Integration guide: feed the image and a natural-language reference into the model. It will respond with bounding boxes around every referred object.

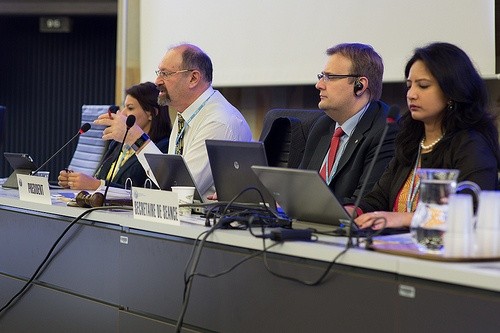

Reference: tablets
[5,152,37,172]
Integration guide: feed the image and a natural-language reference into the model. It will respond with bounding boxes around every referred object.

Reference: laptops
[251,165,410,238]
[143,152,220,212]
[205,140,288,219]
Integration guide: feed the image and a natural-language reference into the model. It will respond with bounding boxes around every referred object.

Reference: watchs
[131,133,149,150]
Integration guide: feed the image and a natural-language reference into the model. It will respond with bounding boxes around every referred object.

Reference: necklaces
[420,136,443,149]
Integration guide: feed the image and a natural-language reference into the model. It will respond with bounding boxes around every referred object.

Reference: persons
[58,83,172,190]
[205,43,402,219]
[92,44,253,199]
[342,43,500,230]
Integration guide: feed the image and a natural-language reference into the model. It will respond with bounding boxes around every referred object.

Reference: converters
[271,228,312,241]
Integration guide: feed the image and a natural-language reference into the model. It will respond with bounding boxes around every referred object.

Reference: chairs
[256,110,328,168]
[67,104,117,176]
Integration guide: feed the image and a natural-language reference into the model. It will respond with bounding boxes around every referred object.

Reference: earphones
[354,83,363,94]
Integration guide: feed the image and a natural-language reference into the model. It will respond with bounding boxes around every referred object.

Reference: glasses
[317,74,369,89]
[155,69,201,80]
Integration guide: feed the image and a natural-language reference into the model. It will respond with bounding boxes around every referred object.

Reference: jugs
[409,167,479,254]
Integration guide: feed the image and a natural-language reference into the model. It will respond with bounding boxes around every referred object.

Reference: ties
[174,114,185,155]
[319,127,345,184]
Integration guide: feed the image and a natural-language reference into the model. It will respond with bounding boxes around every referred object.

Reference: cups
[170,186,195,217]
[442,190,500,261]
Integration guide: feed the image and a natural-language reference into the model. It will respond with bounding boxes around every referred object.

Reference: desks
[0,177,500,333]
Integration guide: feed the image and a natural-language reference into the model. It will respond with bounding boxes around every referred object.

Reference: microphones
[32,122,92,176]
[103,114,136,207]
